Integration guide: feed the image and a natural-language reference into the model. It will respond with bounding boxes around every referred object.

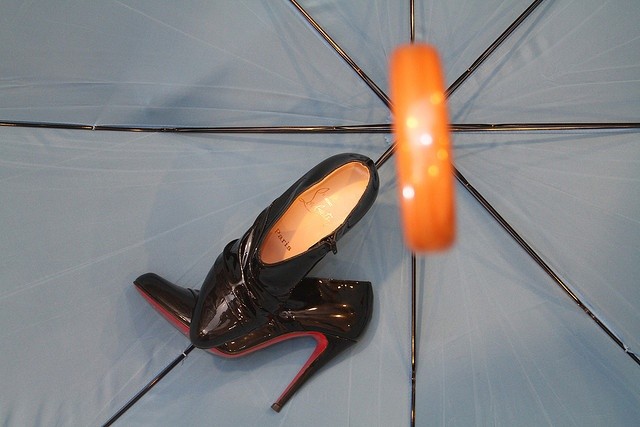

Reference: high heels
[189,153,379,348]
[132,272,373,413]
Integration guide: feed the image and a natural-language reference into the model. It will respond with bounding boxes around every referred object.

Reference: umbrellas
[0,0,640,427]
